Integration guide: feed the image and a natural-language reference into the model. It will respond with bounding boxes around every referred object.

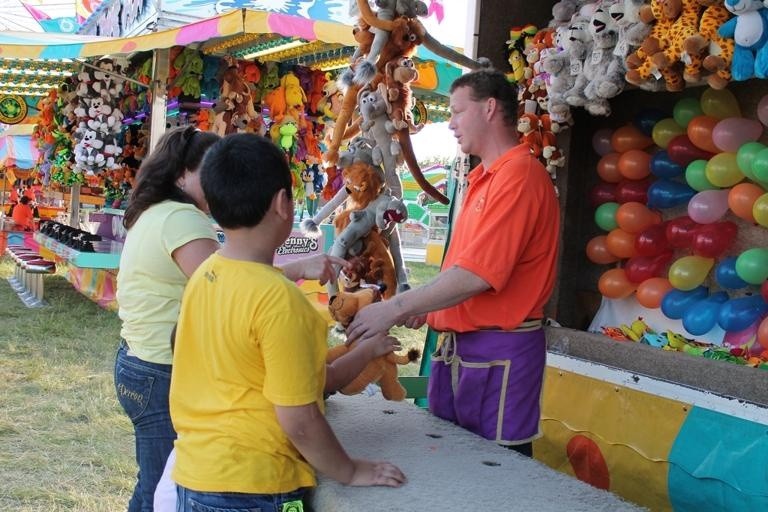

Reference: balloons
[580,87,767,366]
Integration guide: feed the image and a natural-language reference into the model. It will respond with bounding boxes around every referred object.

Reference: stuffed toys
[1,0,768,401]
[300,0,476,400]
[503,2,768,198]
[0,60,344,254]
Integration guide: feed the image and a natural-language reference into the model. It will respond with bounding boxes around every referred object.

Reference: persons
[343,73,562,459]
[115,125,408,512]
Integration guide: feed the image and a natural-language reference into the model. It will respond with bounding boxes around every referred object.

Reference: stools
[5,245,54,310]
